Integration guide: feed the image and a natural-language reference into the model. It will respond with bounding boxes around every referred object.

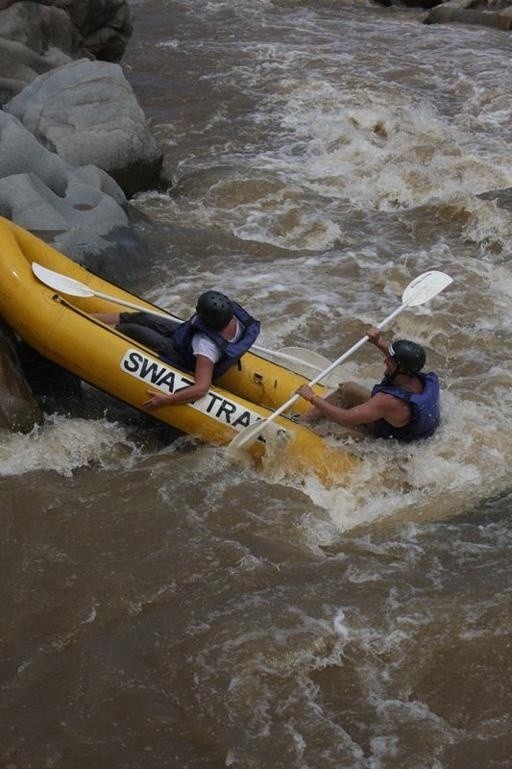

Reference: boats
[0,214,362,484]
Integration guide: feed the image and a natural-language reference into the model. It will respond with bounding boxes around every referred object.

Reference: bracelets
[310,394,319,403]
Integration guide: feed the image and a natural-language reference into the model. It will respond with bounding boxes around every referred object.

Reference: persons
[295,326,439,443]
[85,291,261,411]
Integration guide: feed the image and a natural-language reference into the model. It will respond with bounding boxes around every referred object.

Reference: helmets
[198,290,235,331]
[388,340,425,376]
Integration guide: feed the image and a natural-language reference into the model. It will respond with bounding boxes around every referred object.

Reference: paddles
[224,273,453,450]
[30,262,333,385]
